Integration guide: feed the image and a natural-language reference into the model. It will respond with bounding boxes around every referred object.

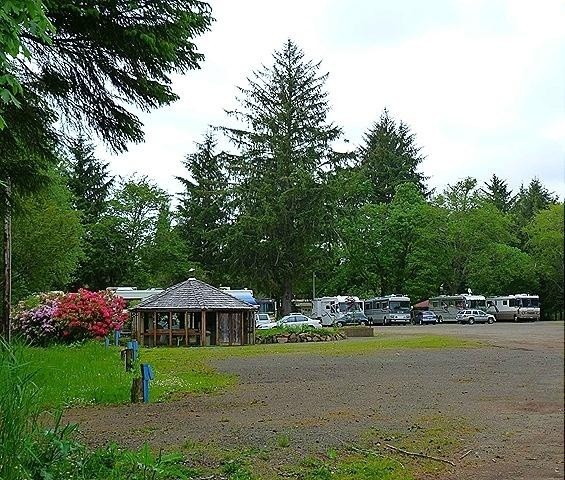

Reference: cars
[255,313,322,330]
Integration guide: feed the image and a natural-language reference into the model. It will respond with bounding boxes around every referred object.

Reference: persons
[172,320,180,329]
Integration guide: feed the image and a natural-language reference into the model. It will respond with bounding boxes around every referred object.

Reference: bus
[313,294,540,327]
